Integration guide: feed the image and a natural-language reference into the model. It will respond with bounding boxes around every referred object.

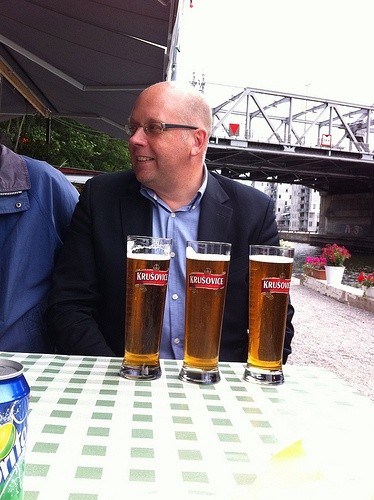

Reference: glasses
[125,123,199,137]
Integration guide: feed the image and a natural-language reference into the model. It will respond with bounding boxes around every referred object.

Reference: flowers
[322,243,351,265]
[302,256,327,270]
[356,271,374,289]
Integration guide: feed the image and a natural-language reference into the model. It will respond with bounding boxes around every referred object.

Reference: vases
[324,265,346,285]
[364,286,374,297]
[304,267,326,280]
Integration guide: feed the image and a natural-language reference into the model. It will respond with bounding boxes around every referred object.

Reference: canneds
[0,359,31,500]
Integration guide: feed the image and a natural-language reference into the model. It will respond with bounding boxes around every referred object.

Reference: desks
[0,352,374,500]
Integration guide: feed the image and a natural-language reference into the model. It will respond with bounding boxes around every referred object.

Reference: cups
[178,241,232,384]
[243,245,294,386]
[118,236,173,381]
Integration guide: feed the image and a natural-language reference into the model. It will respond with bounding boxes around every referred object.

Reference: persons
[0,144,82,354]
[44,81,295,365]
